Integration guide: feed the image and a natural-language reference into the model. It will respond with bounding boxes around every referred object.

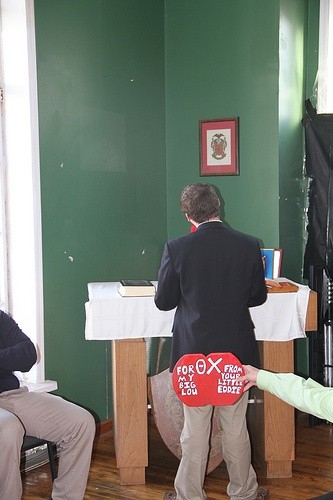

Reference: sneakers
[256,486,270,500]
[164,490,176,500]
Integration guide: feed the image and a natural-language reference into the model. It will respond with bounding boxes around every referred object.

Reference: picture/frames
[199,116,240,178]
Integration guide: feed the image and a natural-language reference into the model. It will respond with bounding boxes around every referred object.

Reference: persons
[233,365,333,500]
[153,183,268,500]
[1,307,96,500]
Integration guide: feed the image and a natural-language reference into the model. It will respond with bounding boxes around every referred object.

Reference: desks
[84,276,317,487]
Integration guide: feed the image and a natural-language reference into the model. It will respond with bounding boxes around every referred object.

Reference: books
[117,279,156,297]
[259,247,283,280]
[265,281,299,294]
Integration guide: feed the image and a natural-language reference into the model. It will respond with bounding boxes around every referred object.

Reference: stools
[18,434,57,482]
